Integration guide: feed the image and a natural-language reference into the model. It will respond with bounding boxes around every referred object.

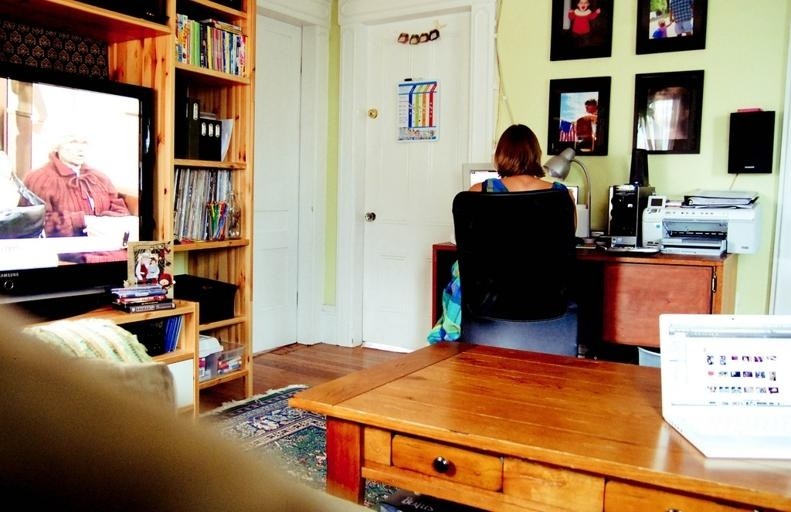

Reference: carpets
[197,381,398,512]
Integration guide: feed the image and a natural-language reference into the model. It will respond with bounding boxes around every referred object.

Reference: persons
[576,100,598,142]
[653,20,674,39]
[568,0,602,35]
[469,123,578,234]
[669,0,694,37]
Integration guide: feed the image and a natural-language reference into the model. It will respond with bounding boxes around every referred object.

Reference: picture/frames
[631,68,705,155]
[546,75,612,157]
[634,0,708,56]
[547,0,614,62]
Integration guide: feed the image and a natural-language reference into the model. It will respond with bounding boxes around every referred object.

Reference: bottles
[227,190,242,239]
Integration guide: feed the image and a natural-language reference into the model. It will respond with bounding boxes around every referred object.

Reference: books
[174,14,246,241]
[16,124,130,237]
[110,283,176,312]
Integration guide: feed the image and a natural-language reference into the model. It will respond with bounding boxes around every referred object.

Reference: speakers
[728,112,775,173]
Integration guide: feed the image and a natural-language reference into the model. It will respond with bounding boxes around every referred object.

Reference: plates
[576,244,597,249]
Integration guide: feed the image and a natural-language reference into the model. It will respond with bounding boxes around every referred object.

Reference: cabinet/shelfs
[22,298,200,421]
[109,0,256,413]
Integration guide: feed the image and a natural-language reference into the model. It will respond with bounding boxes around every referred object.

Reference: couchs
[0,315,376,512]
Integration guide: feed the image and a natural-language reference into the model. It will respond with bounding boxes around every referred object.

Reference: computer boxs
[607,186,655,248]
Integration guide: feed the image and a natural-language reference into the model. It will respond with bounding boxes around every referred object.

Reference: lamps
[543,146,592,237]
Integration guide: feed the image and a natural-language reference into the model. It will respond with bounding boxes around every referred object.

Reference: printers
[643,195,761,258]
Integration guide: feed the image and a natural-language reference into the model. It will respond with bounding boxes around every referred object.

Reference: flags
[559,121,575,142]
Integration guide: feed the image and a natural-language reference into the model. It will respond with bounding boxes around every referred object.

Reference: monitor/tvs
[0,64,155,297]
[470,170,501,185]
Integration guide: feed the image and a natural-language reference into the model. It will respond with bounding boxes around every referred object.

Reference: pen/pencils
[205,197,230,242]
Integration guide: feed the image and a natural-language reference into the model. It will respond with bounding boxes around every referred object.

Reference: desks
[430,240,738,348]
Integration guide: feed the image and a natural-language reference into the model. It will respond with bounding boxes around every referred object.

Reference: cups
[584,231,611,247]
[174,211,185,243]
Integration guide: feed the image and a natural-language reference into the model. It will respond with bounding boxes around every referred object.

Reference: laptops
[659,314,791,460]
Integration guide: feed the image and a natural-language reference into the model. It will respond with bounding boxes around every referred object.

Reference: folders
[680,187,760,208]
[174,97,200,160]
